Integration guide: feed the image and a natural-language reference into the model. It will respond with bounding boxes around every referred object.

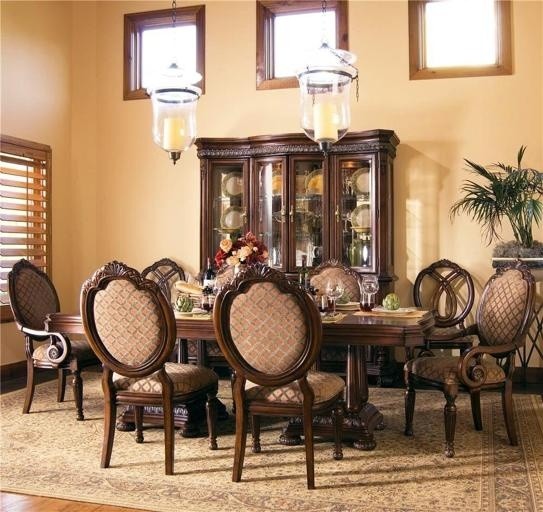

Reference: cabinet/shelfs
[195,129,400,385]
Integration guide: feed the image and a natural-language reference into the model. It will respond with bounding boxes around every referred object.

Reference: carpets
[0,392,542,512]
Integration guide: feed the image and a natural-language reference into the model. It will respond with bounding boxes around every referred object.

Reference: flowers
[214,228,268,268]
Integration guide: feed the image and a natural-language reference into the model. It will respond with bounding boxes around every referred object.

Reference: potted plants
[448,145,543,269]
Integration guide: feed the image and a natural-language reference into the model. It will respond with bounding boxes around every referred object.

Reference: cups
[324,276,345,319]
[360,273,380,312]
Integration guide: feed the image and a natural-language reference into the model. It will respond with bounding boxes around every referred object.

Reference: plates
[350,226,370,234]
[219,205,244,234]
[271,170,282,195]
[335,301,360,309]
[349,204,374,233]
[220,170,245,200]
[173,308,208,319]
[303,168,323,196]
[295,170,310,198]
[348,167,370,198]
[370,307,419,318]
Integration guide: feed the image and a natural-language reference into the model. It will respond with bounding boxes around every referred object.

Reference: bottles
[202,257,216,309]
[299,255,310,289]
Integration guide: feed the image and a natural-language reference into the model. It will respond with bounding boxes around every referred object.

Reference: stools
[496,269,543,391]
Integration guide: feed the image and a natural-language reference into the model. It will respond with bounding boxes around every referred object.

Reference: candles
[314,101,338,143]
[164,113,186,147]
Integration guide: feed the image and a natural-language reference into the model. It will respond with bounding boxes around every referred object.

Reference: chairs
[214,266,345,488]
[306,260,364,302]
[79,260,218,476]
[142,257,187,305]
[7,258,94,420]
[406,260,480,357]
[404,259,536,458]
